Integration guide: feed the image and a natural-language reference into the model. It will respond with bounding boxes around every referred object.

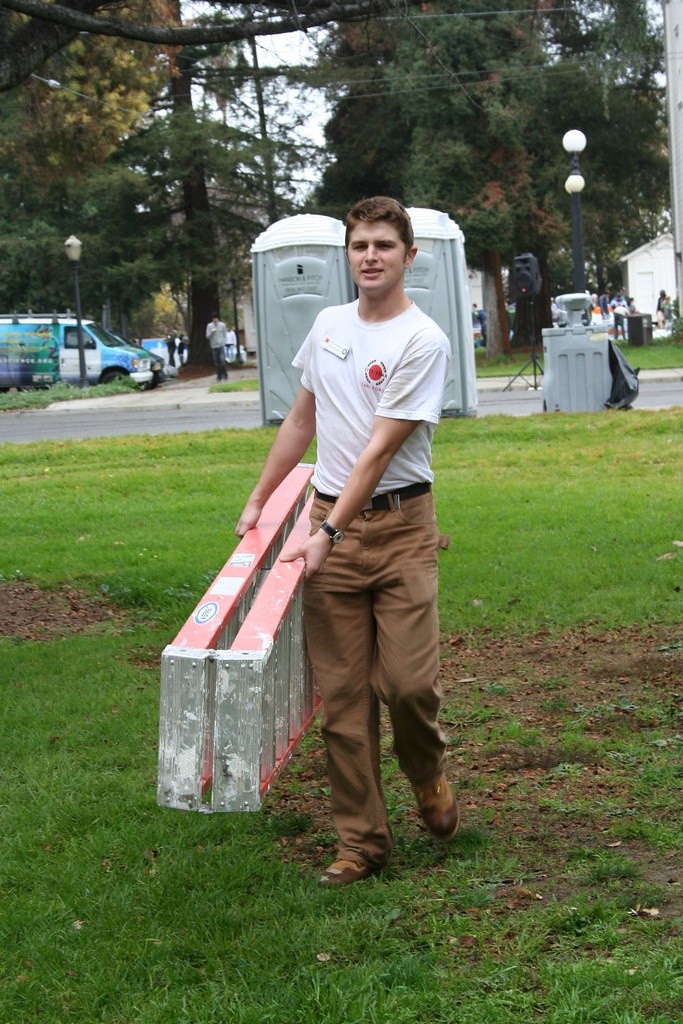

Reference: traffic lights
[514,252,539,301]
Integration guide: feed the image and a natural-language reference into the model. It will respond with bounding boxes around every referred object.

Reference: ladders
[156,464,324,813]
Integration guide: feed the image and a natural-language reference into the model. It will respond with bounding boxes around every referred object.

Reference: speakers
[513,254,542,297]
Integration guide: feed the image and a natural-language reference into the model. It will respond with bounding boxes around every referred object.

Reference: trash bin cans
[541,293,612,413]
[627,314,653,346]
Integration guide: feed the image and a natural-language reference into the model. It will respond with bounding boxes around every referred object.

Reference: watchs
[319,520,344,544]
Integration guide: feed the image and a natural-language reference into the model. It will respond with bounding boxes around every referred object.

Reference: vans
[0,309,178,392]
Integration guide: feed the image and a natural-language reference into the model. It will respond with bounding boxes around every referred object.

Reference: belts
[315,482,429,511]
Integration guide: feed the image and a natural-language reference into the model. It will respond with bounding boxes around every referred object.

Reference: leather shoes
[414,773,461,842]
[319,858,377,889]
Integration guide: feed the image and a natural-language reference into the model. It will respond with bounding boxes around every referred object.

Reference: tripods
[501,298,544,392]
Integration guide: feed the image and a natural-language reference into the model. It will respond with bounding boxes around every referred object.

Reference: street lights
[65,234,89,391]
[557,128,594,298]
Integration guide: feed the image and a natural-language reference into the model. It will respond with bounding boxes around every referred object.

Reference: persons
[551,298,561,328]
[178,334,187,365]
[235,196,460,890]
[163,325,177,367]
[205,311,228,381]
[504,299,516,329]
[474,308,487,349]
[224,324,236,360]
[587,285,640,341]
[657,289,674,335]
